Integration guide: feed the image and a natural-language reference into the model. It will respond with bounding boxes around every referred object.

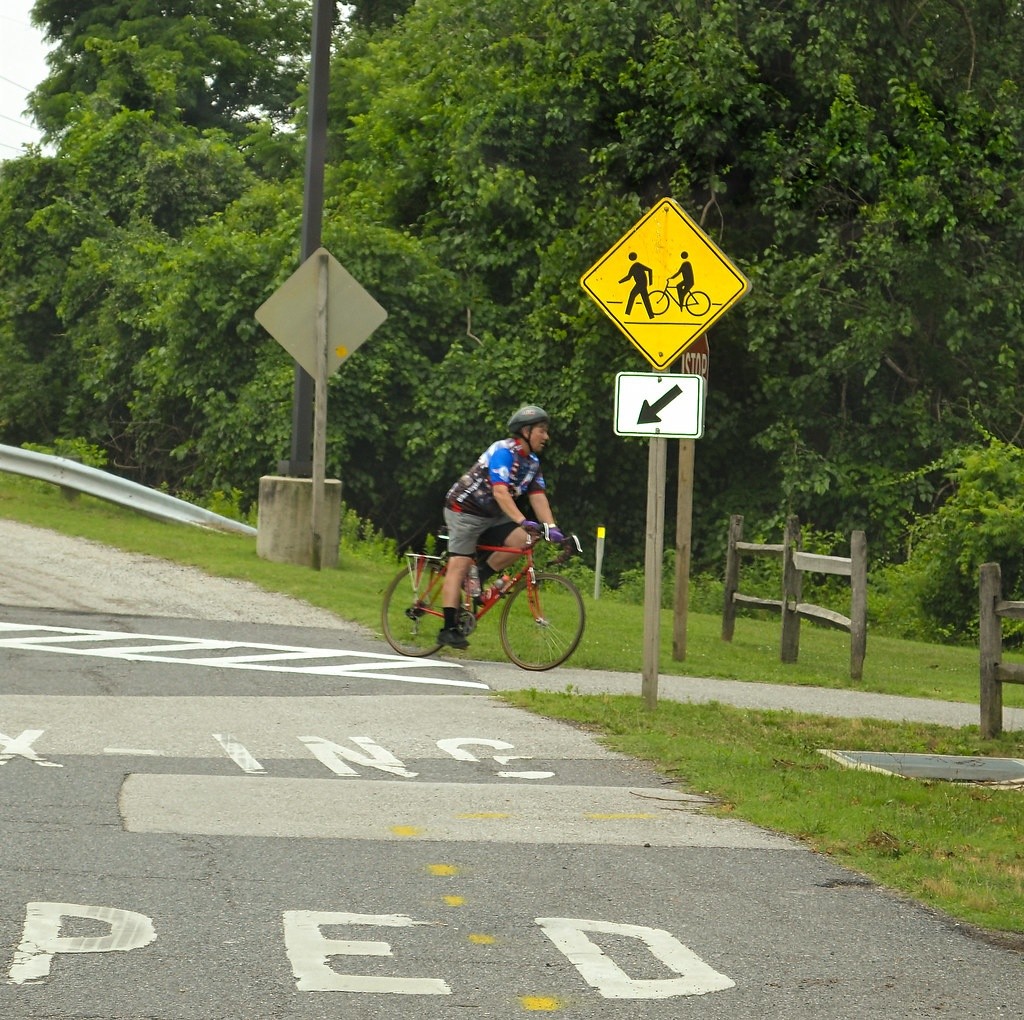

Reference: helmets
[508,406,549,433]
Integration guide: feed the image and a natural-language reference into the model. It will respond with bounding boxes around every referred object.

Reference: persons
[436,406,564,649]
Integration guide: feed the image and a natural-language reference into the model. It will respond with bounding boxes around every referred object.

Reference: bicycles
[381,519,584,671]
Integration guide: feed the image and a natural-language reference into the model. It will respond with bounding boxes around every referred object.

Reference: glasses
[529,427,548,432]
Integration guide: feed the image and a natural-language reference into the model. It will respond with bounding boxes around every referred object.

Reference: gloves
[520,519,542,537]
[550,525,563,544]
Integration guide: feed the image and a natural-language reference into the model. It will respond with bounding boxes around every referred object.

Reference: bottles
[477,576,510,609]
[466,559,481,598]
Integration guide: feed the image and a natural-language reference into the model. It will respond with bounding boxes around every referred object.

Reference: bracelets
[549,524,556,528]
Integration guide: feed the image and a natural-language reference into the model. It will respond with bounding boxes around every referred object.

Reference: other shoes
[437,628,470,648]
[461,580,484,605]
